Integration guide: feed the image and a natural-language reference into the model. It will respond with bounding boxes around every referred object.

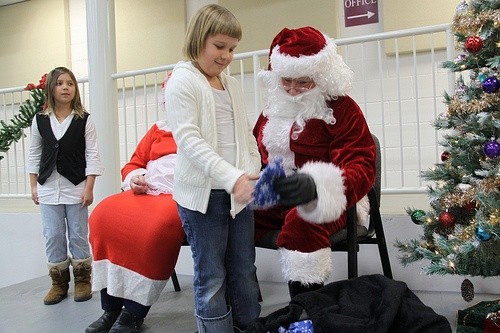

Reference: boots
[43,255,71,305]
[85,287,152,333]
[71,255,93,301]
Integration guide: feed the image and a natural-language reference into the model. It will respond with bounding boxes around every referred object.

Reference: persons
[252,26,377,300]
[85,69,189,333]
[164,4,261,333]
[27,67,103,305]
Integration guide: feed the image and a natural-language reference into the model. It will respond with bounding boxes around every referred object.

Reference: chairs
[170,135,393,302]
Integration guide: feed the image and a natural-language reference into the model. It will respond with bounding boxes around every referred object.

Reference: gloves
[274,171,316,208]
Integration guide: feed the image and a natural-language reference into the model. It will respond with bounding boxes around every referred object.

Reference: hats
[267,24,337,78]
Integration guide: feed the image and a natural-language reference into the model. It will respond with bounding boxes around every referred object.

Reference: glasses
[276,81,313,95]
